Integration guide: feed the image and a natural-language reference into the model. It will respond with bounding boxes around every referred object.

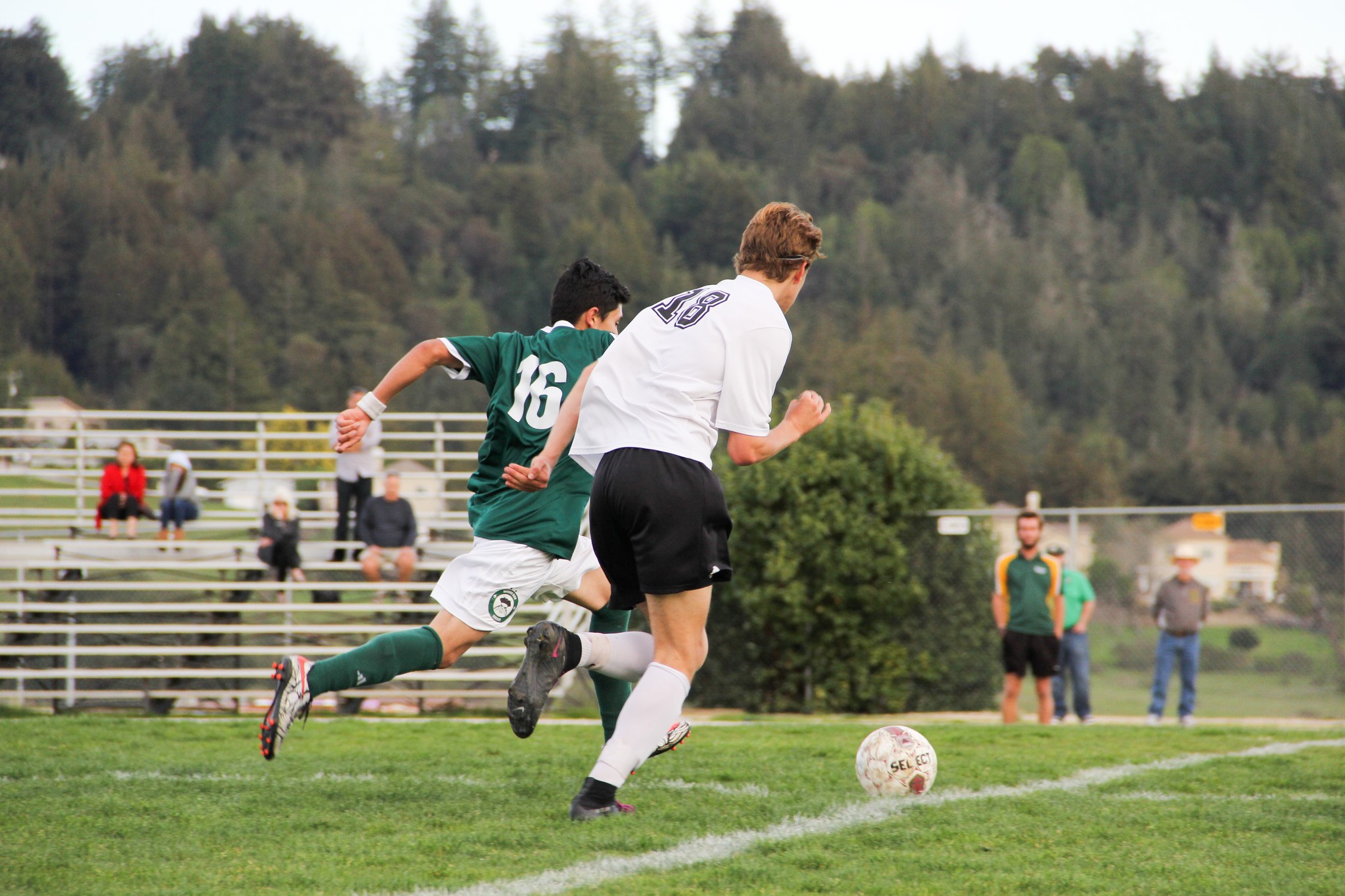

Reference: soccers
[855,724,937,798]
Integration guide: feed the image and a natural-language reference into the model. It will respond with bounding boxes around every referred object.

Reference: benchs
[1,408,577,715]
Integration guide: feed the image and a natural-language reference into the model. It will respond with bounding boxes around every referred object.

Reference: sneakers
[629,721,693,776]
[259,653,317,761]
[571,794,636,824]
[506,619,571,739]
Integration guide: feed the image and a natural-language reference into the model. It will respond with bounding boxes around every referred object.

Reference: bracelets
[355,390,389,422]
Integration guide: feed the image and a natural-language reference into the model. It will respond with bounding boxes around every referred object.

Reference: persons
[1042,538,1098,727]
[355,471,420,600]
[323,385,383,565]
[255,491,308,605]
[94,441,148,538]
[989,512,1065,725]
[153,448,200,553]
[1142,549,1211,726]
[507,198,832,820]
[261,255,690,768]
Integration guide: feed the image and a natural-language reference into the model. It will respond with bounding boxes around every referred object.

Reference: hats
[1047,546,1062,554]
[1171,545,1198,566]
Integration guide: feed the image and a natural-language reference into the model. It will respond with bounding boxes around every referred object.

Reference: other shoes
[1182,714,1195,728]
[1144,713,1159,728]
